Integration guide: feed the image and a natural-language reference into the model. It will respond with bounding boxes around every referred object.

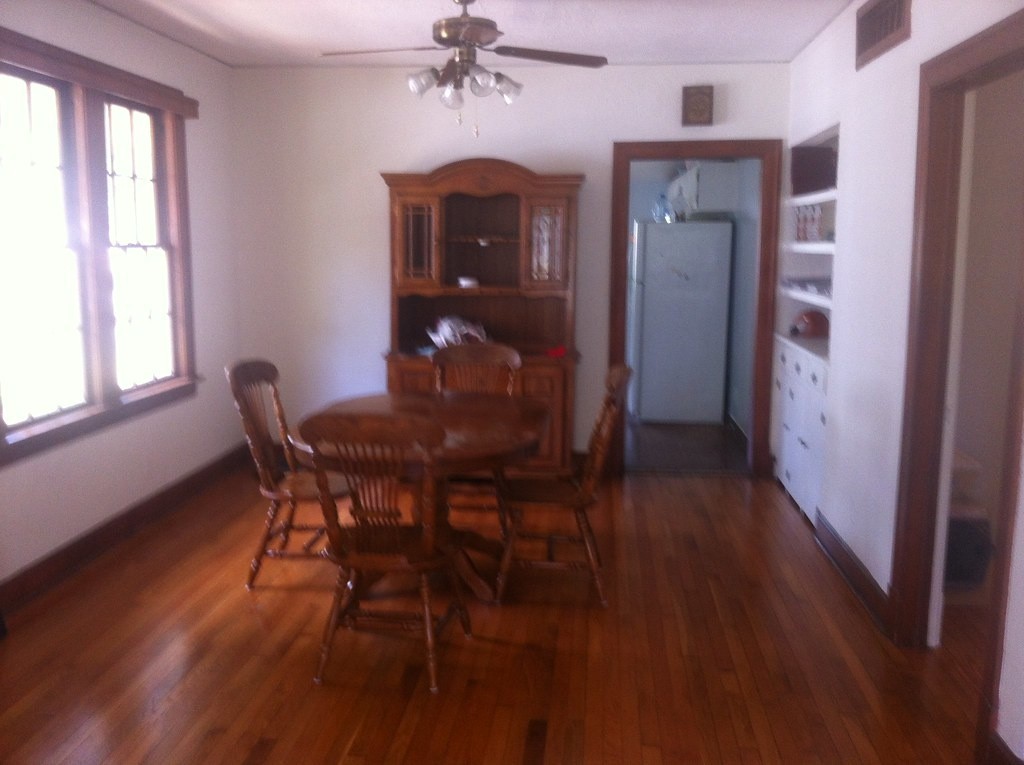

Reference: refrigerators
[633,220,732,423]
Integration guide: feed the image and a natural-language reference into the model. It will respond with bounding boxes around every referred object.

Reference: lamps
[405,45,525,109]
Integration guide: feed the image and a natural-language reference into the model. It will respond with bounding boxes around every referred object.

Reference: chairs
[297,415,472,696]
[494,362,634,608]
[431,347,521,542]
[225,357,359,592]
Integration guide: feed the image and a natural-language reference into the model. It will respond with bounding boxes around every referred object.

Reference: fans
[319,0,608,108]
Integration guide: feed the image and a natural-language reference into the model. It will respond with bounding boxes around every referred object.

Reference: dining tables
[285,391,538,603]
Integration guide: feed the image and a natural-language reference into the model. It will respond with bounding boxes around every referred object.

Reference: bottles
[652,195,672,222]
[673,185,688,221]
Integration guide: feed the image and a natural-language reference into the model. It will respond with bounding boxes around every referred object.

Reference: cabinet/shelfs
[770,186,838,527]
[378,157,587,484]
